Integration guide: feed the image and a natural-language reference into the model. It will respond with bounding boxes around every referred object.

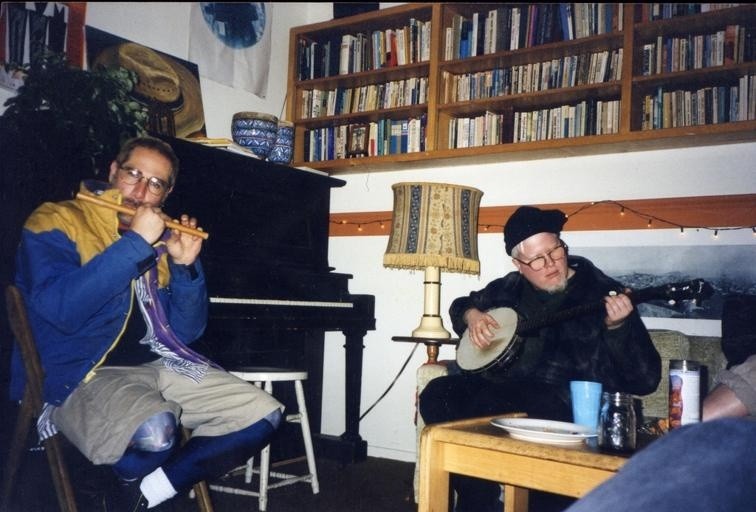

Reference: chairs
[0,283,215,511]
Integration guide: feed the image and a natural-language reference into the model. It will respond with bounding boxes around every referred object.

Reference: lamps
[383,181,486,339]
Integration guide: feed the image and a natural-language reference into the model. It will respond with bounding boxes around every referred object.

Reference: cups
[570,380,603,439]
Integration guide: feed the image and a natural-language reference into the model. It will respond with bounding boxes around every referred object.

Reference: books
[634,1,756,130]
[297,17,431,162]
[443,2,623,148]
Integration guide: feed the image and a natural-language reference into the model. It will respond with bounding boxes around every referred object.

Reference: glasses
[514,239,568,272]
[119,166,170,195]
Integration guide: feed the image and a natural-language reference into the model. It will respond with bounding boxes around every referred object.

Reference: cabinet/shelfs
[284,3,756,175]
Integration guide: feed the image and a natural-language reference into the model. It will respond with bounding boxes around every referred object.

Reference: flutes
[76,193,209,239]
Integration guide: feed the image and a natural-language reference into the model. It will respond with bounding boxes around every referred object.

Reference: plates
[489,418,600,446]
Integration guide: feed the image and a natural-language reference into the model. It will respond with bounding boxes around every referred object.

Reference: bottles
[598,392,637,453]
[667,358,702,432]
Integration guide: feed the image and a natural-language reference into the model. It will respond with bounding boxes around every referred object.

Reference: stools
[189,366,321,511]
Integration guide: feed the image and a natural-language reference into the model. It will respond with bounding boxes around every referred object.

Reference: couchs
[412,328,729,504]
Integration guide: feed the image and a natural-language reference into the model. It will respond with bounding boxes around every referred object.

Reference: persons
[695,350,755,424]
[9,137,286,512]
[417,204,663,512]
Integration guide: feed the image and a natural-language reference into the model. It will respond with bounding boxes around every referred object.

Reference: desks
[391,335,460,504]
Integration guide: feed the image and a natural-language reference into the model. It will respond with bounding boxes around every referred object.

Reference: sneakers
[102,476,148,512]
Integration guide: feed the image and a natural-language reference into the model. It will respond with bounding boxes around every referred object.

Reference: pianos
[1,110,374,512]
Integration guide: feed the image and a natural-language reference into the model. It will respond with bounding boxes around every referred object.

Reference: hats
[504,206,568,256]
